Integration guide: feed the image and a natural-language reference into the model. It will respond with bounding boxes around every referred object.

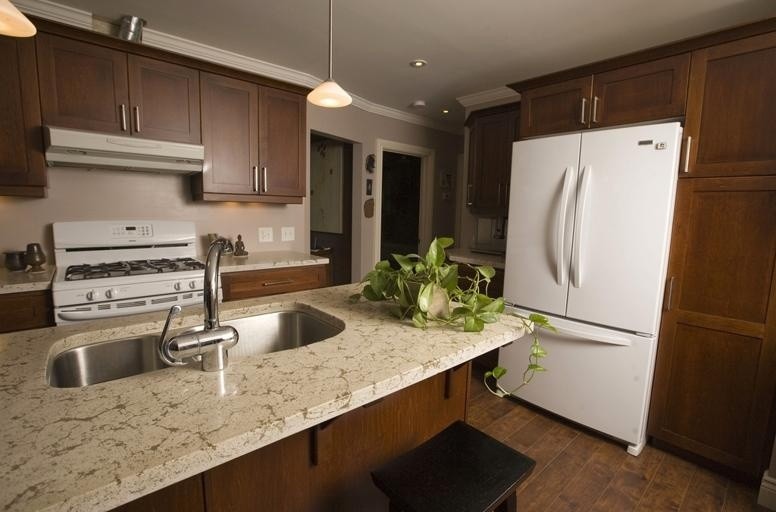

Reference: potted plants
[344,233,558,398]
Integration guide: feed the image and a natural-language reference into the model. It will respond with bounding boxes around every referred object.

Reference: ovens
[53,288,223,326]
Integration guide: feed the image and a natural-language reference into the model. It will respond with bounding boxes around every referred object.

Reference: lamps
[305,0,354,108]
[0,0,40,40]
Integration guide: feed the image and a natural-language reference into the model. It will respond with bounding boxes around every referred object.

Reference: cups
[2,250,27,272]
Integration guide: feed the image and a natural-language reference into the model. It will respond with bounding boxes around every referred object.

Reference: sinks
[48,333,184,389]
[188,300,346,359]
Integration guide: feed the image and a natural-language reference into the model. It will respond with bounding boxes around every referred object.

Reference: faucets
[156,239,240,371]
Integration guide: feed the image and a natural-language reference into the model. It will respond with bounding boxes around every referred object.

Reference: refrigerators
[491,117,685,465]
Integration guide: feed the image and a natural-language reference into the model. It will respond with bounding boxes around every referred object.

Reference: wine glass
[24,243,46,272]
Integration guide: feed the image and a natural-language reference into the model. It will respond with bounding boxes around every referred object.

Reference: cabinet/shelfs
[35,15,201,147]
[505,38,693,140]
[0,289,56,334]
[190,57,313,207]
[462,99,521,220]
[220,264,334,303]
[644,15,776,177]
[646,166,776,487]
[0,11,50,199]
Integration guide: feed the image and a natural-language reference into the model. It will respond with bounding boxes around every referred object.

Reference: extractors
[45,124,204,179]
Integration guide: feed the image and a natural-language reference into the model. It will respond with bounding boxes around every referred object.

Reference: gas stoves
[64,256,205,282]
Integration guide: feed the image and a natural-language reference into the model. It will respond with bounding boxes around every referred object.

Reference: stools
[366,417,539,511]
